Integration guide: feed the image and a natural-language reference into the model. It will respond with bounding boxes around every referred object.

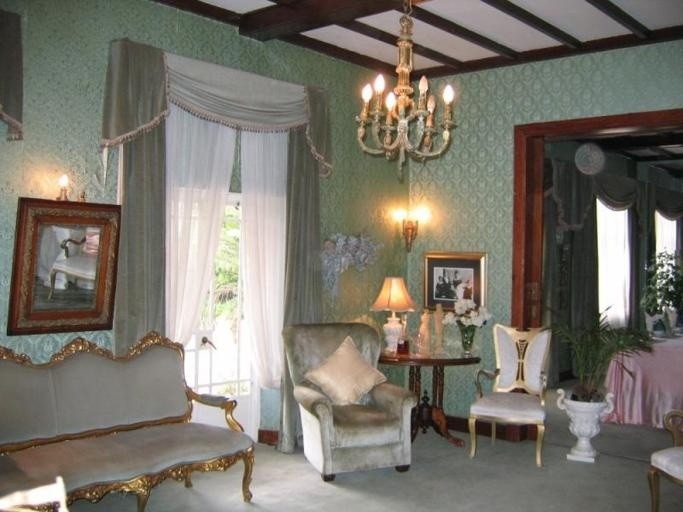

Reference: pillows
[302,335,387,407]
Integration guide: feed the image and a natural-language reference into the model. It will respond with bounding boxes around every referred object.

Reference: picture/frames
[424,251,488,317]
[8,197,121,334]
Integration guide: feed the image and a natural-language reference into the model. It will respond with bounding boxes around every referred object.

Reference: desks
[376,353,481,447]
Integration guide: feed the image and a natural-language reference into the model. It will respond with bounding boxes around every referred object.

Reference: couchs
[0,331,255,512]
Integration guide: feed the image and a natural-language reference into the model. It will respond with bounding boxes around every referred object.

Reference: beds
[600,334,683,430]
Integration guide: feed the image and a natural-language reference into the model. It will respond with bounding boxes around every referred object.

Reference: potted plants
[640,245,683,331]
[541,308,662,467]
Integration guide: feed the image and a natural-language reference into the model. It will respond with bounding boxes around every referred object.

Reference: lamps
[385,204,430,252]
[56,174,73,200]
[354,0,457,180]
[370,276,418,353]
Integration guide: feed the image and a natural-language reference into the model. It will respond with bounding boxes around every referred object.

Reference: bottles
[396,315,411,358]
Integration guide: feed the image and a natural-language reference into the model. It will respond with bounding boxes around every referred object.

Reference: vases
[459,324,477,357]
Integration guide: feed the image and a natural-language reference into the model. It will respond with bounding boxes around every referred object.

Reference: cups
[383,340,397,358]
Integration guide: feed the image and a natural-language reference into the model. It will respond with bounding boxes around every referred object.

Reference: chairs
[648,408,683,512]
[282,323,419,481]
[48,236,101,300]
[468,322,552,468]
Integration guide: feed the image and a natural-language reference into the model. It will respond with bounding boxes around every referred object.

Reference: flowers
[319,231,383,305]
[442,298,493,328]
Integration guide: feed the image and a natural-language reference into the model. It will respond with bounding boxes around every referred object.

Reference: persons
[434,269,473,300]
